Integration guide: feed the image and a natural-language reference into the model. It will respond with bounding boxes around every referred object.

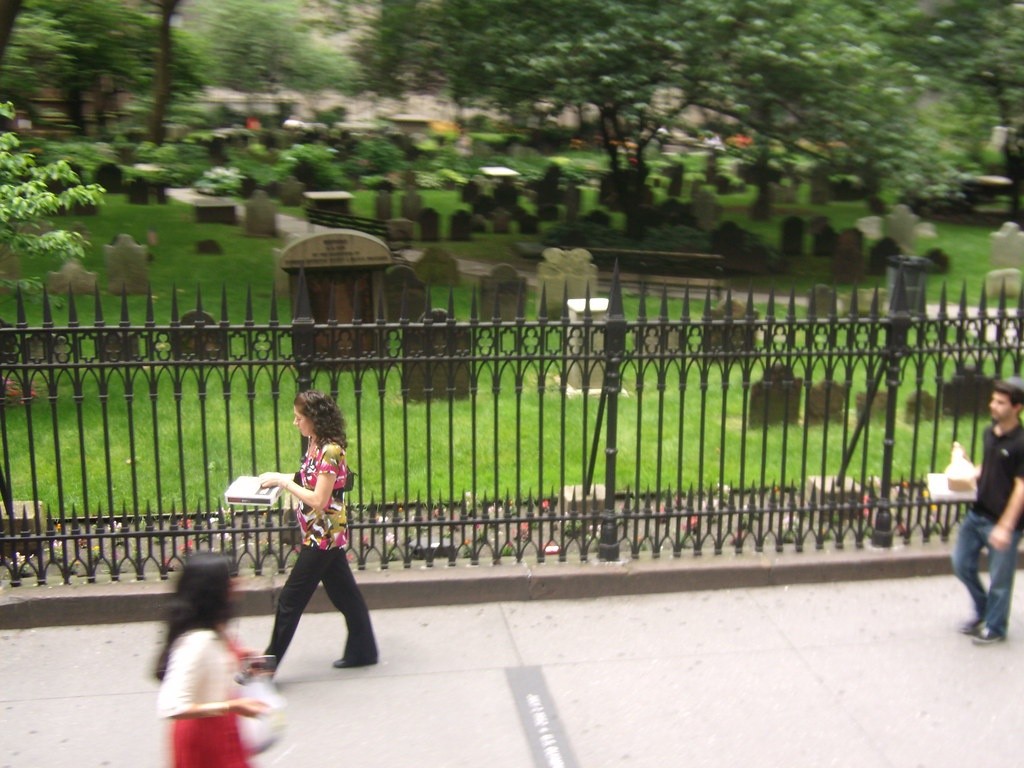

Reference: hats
[994,376,1024,392]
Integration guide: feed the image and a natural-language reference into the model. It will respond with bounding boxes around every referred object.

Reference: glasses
[300,456,307,465]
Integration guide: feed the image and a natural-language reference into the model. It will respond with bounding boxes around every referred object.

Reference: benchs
[588,247,727,301]
[303,205,413,259]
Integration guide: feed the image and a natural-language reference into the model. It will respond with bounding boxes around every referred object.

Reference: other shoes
[333,658,378,668]
[961,616,988,633]
[250,661,276,681]
[973,623,1006,643]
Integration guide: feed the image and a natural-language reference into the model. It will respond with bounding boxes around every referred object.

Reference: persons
[153,551,275,768]
[258,392,379,668]
[951,377,1024,644]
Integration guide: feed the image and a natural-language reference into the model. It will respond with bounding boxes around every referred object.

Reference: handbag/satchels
[293,465,358,491]
[222,629,292,757]
